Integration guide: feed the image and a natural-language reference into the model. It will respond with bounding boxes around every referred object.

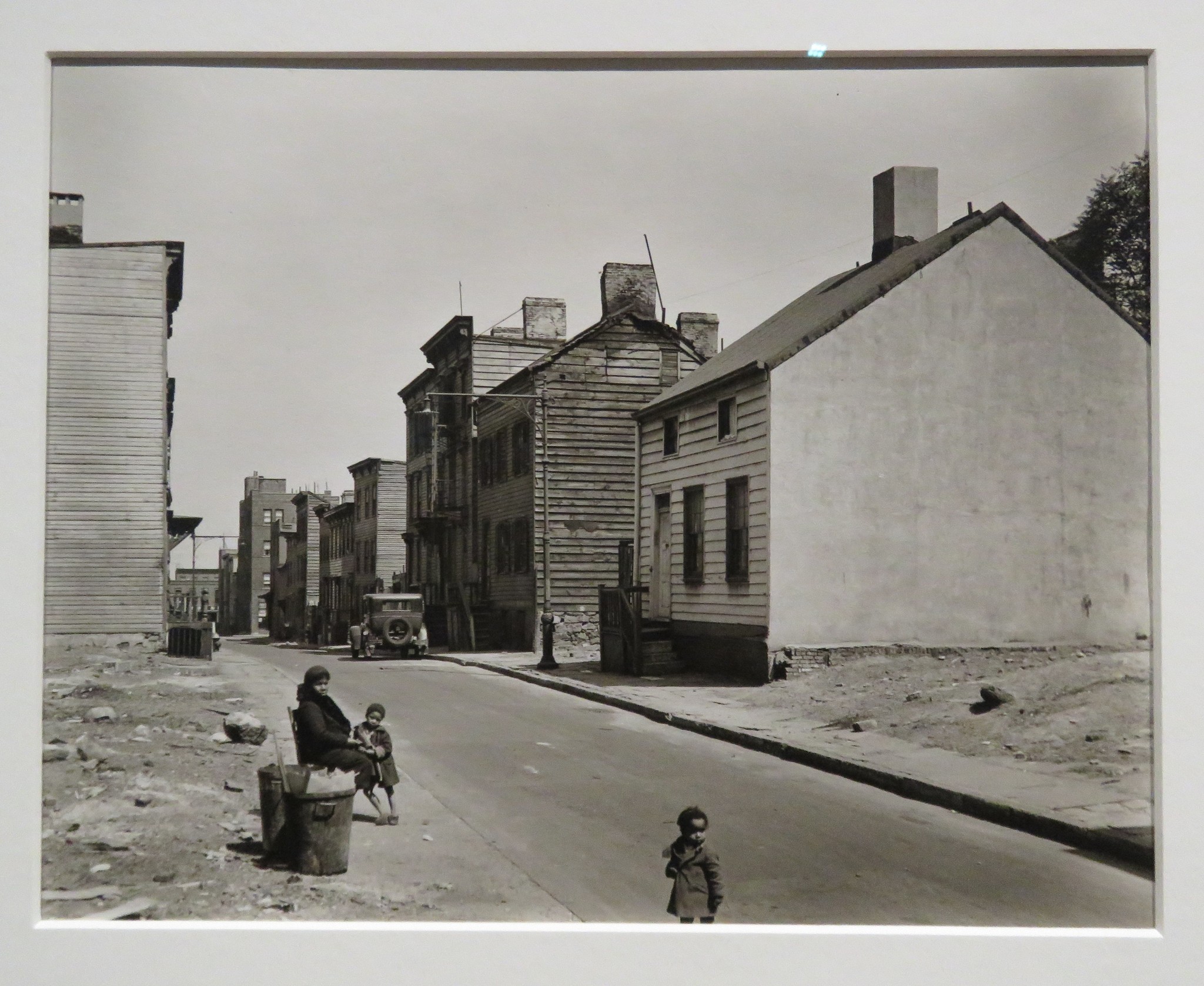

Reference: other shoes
[388,815,399,825]
[376,813,388,826]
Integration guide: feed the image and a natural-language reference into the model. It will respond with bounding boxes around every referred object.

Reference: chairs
[286,705,327,770]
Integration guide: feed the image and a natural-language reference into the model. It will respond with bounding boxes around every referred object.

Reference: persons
[665,806,724,924]
[295,664,373,796]
[352,703,400,826]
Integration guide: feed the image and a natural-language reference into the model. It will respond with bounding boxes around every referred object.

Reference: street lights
[414,373,561,671]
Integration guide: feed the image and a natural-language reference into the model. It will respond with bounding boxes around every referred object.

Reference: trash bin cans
[281,784,357,876]
[258,764,328,864]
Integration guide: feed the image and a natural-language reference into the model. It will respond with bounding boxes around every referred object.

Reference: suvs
[348,593,429,660]
